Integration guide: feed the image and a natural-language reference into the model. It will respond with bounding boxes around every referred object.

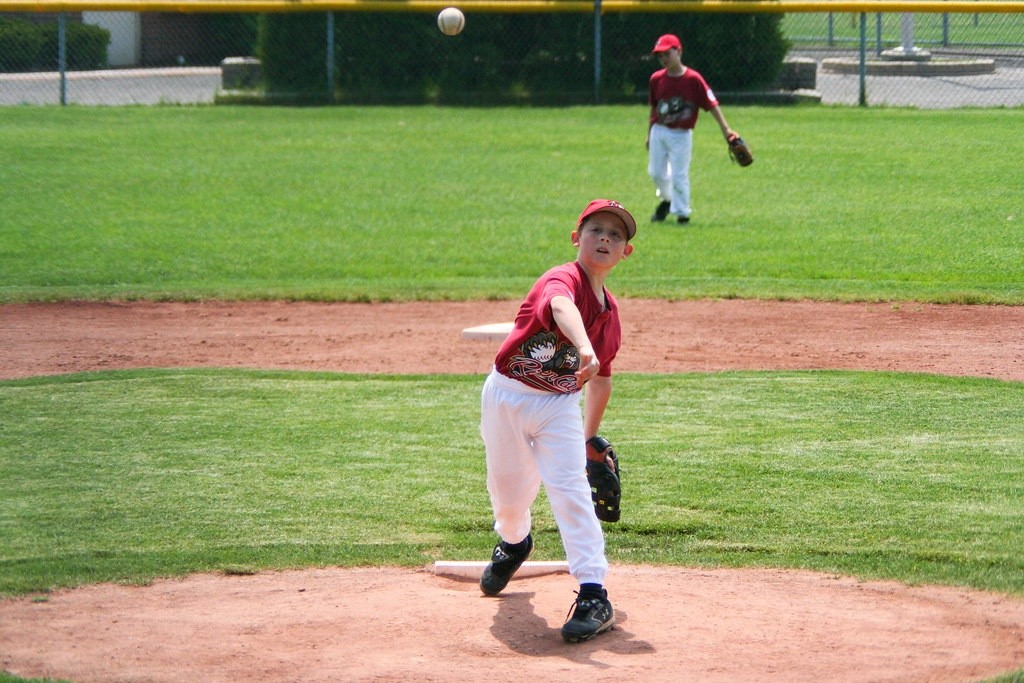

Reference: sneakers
[560,587,616,643]
[480,533,535,596]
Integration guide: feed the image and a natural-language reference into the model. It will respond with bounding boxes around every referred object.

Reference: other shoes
[650,200,671,221]
[677,217,688,226]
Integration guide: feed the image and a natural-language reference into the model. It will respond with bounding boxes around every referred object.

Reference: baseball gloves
[726,134,754,167]
[585,435,622,523]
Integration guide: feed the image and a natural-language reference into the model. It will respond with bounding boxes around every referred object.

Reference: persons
[645,34,753,223]
[480,199,636,643]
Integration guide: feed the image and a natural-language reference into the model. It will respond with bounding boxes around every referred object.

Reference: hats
[652,34,682,53]
[578,200,636,241]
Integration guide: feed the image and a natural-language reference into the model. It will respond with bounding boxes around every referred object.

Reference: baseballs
[437,7,466,36]
